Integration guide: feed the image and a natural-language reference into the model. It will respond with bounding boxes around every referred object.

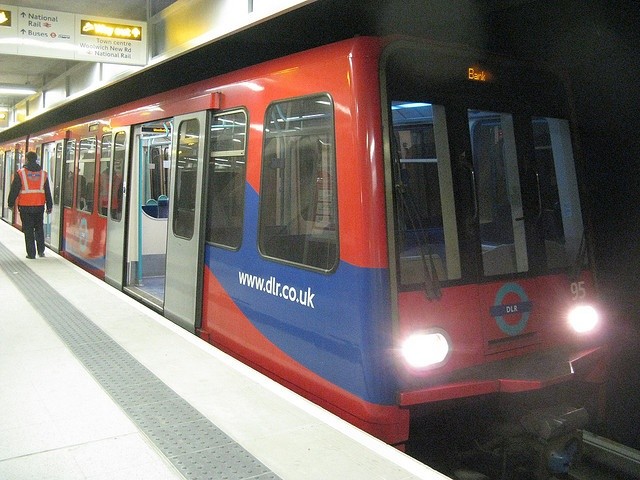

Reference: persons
[99,160,122,220]
[8,152,52,259]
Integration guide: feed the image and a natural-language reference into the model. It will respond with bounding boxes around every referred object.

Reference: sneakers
[38,252,45,256]
[26,255,35,259]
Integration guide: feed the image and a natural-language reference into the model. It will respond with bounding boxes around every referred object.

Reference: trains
[1,37,607,480]
